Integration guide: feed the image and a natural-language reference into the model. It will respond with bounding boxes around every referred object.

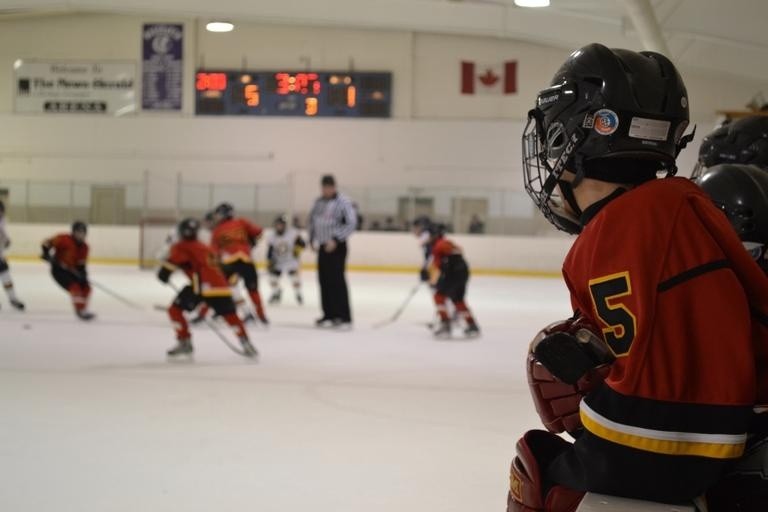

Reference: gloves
[507,430,585,512]
[527,317,610,440]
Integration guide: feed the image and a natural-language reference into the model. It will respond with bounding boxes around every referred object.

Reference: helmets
[522,44,697,235]
[690,116,767,252]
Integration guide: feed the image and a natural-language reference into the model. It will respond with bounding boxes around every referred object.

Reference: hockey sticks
[40,252,154,313]
[371,277,419,330]
[167,278,247,355]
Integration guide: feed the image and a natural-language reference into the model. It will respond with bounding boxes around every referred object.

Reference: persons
[508,43,768,512]
[41,222,96,319]
[688,102,768,277]
[419,223,482,340]
[1,202,26,311]
[155,199,269,360]
[308,176,357,328]
[264,213,307,305]
[413,214,462,328]
[291,203,488,235]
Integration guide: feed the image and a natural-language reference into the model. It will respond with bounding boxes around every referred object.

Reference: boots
[463,323,481,332]
[240,341,258,354]
[167,338,193,355]
[435,320,452,333]
